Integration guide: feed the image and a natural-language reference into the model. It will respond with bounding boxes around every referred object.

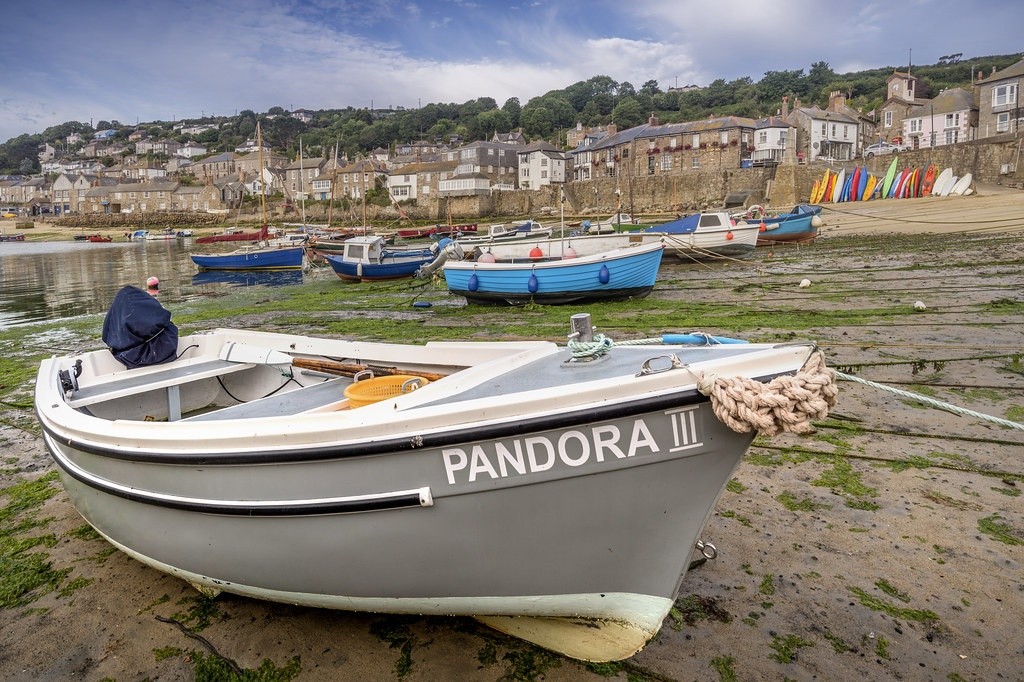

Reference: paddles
[219,342,443,392]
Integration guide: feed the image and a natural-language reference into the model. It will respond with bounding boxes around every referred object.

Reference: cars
[862,142,909,158]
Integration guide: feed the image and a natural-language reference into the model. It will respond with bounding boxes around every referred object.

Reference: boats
[73,228,113,242]
[34,286,817,661]
[810,156,973,204]
[124,224,193,240]
[611,204,823,258]
[0,228,26,242]
[189,122,667,309]
[565,221,582,227]
[588,214,640,235]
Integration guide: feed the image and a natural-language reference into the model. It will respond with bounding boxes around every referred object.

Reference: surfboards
[809,156,975,205]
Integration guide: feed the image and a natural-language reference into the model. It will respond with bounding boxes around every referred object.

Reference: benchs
[63,356,363,425]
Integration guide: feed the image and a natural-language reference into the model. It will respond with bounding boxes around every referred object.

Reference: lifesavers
[746,205,765,219]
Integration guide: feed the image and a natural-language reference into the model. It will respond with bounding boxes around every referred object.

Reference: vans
[1,207,25,217]
